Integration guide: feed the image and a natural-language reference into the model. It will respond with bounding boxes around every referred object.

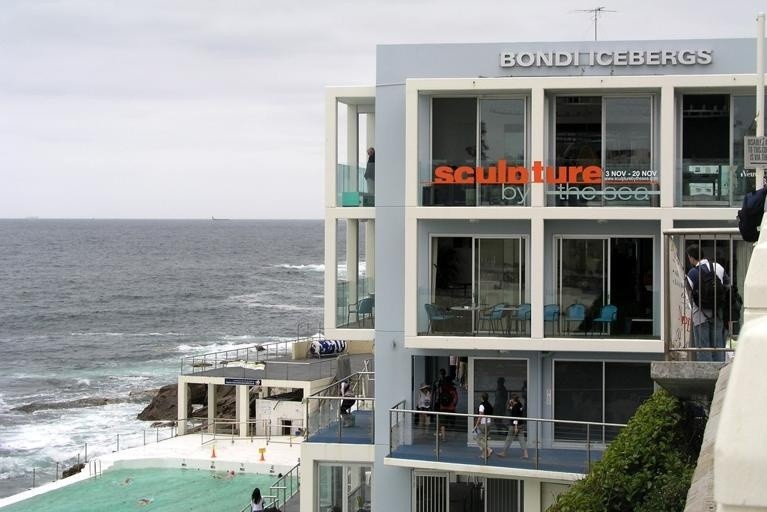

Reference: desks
[498,308,521,337]
[451,306,476,336]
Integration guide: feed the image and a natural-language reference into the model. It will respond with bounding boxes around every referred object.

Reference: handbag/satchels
[720,271,742,335]
[736,187,766,242]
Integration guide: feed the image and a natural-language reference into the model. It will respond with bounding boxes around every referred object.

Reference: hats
[418,383,431,390]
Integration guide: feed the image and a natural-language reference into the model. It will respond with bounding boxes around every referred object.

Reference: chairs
[562,304,586,336]
[347,298,374,328]
[425,304,448,335]
[506,304,531,336]
[544,304,559,336]
[477,303,505,335]
[591,305,618,336]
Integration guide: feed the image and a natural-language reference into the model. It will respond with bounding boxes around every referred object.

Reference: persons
[562,129,597,207]
[249,486,265,512]
[414,355,529,459]
[362,147,374,195]
[336,377,355,428]
[681,242,732,362]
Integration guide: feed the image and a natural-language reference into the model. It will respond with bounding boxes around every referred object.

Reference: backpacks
[438,390,453,408]
[686,259,724,319]
